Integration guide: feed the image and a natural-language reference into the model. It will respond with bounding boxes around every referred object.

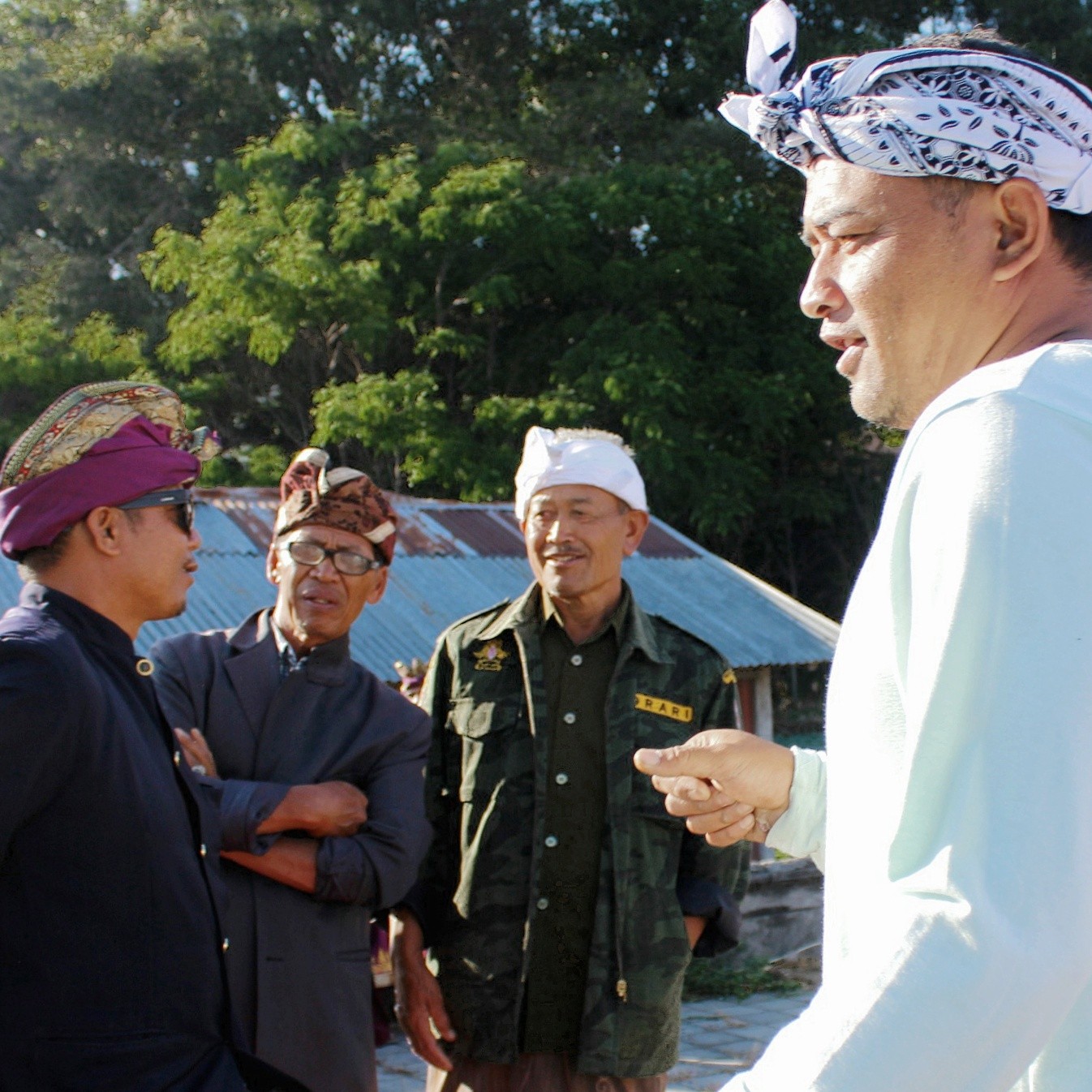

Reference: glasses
[116,488,194,534]
[273,540,385,576]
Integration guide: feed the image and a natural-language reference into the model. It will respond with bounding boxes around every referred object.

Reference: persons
[388,423,751,1091]
[140,442,431,1092]
[1,374,240,1092]
[634,0,1092,1092]
[386,655,430,705]
